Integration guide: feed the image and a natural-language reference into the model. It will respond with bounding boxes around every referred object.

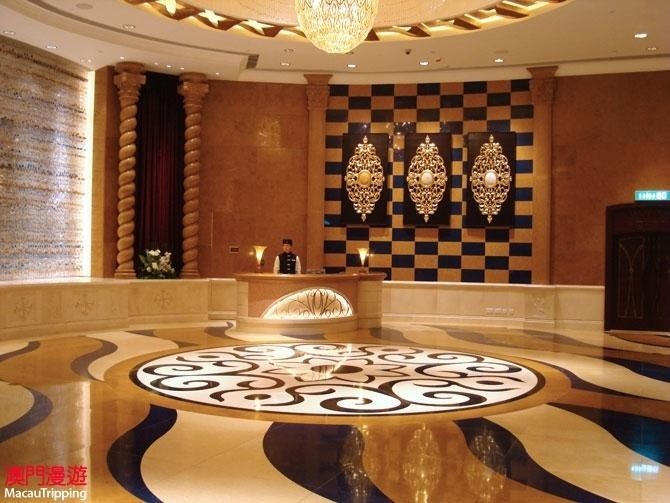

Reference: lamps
[295,0,379,55]
[256,288,354,321]
[356,247,370,274]
[252,244,268,271]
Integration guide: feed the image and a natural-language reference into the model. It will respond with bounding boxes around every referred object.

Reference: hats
[283,239,292,244]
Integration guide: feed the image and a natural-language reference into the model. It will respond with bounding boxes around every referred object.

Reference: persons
[273,239,301,274]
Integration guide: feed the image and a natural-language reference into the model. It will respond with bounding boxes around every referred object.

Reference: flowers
[138,246,178,278]
